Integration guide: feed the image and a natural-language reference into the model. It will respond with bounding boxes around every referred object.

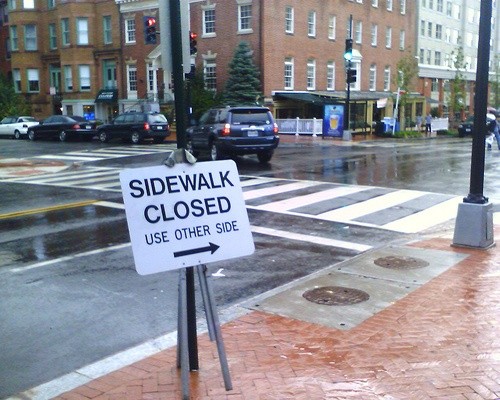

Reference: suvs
[95,110,170,144]
[185,103,280,162]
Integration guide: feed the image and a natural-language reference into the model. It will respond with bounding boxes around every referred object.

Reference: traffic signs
[119,158,256,277]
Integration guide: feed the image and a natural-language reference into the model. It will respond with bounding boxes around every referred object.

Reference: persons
[415,111,423,132]
[424,112,432,134]
[486,112,500,150]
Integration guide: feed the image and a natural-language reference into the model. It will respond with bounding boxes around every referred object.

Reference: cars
[0,115,40,139]
[458,113,495,136]
[27,114,98,141]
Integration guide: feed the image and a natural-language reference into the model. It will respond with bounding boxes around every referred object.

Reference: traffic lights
[347,69,357,84]
[146,17,156,41]
[189,31,197,55]
[344,38,354,61]
